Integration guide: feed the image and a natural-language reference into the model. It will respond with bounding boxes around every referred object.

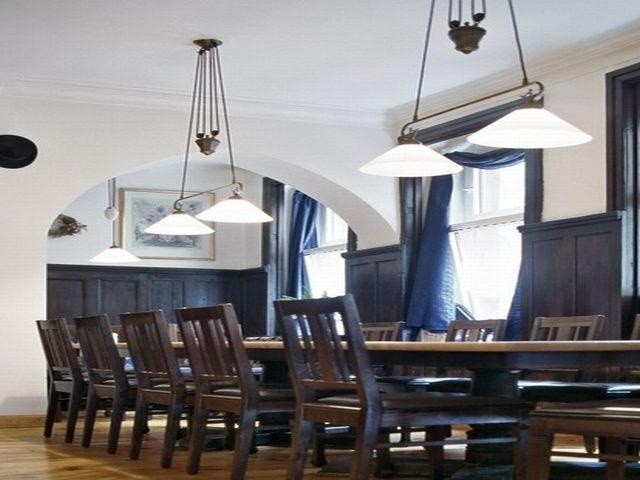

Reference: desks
[62,328,640,468]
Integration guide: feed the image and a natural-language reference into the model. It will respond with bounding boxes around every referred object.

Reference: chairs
[276,295,514,478]
[518,374,640,480]
[73,313,136,453]
[170,301,299,470]
[631,314,640,340]
[530,314,605,343]
[120,310,202,467]
[37,318,90,445]
[358,321,405,343]
[167,319,318,341]
[446,318,504,343]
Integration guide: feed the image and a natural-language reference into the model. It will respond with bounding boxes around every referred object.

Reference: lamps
[144,39,274,236]
[90,178,141,263]
[358,0,592,178]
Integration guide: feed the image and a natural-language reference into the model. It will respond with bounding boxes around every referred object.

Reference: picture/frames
[119,188,215,261]
